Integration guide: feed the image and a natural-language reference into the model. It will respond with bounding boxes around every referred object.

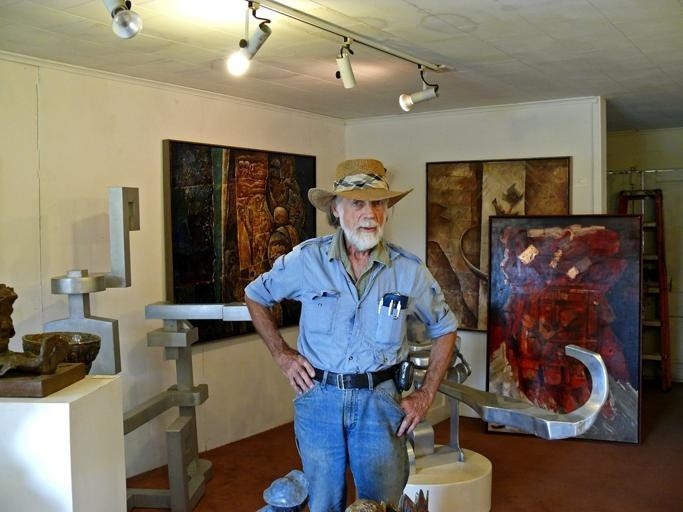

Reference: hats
[307,158,414,215]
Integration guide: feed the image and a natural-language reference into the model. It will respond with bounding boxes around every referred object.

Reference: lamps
[241,0,458,113]
[98,0,143,40]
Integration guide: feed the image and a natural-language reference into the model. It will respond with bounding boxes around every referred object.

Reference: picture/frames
[485,213,641,445]
[425,157,573,336]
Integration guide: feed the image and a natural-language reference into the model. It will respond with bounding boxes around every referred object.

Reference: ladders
[615,189,673,392]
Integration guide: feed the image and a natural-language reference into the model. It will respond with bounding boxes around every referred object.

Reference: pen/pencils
[378,297,402,318]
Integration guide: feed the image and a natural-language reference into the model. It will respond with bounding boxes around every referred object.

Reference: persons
[241,157,461,512]
[266,206,298,264]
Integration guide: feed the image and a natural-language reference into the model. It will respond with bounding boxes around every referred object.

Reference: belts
[312,362,401,389]
